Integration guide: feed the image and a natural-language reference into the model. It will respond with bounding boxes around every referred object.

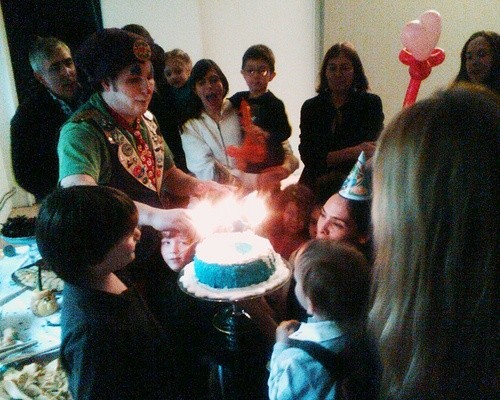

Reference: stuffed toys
[225,99,269,173]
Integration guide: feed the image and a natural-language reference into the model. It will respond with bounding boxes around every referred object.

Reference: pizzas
[15,266,65,292]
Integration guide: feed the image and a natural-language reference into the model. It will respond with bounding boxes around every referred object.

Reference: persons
[120,24,153,50]
[263,184,314,321]
[453,30,500,94]
[177,59,299,190]
[221,45,291,192]
[149,48,200,173]
[277,177,344,328]
[11,37,92,205]
[35,186,176,400]
[266,238,383,400]
[365,80,500,400]
[152,63,175,112]
[298,42,383,189]
[141,220,223,399]
[238,192,372,342]
[58,28,238,231]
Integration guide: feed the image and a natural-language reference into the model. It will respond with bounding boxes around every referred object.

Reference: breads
[30,289,59,317]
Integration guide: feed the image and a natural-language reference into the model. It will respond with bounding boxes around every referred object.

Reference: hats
[77,27,152,84]
[339,150,372,201]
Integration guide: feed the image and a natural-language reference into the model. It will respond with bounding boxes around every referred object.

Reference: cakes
[179,232,290,301]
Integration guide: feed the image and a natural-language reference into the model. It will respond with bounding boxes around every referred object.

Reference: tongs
[0,340,38,360]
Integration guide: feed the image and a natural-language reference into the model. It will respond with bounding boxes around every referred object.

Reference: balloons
[399,9,446,112]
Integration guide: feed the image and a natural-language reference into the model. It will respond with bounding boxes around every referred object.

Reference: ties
[113,113,157,187]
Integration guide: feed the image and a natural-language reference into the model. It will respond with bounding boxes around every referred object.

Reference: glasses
[245,68,268,78]
